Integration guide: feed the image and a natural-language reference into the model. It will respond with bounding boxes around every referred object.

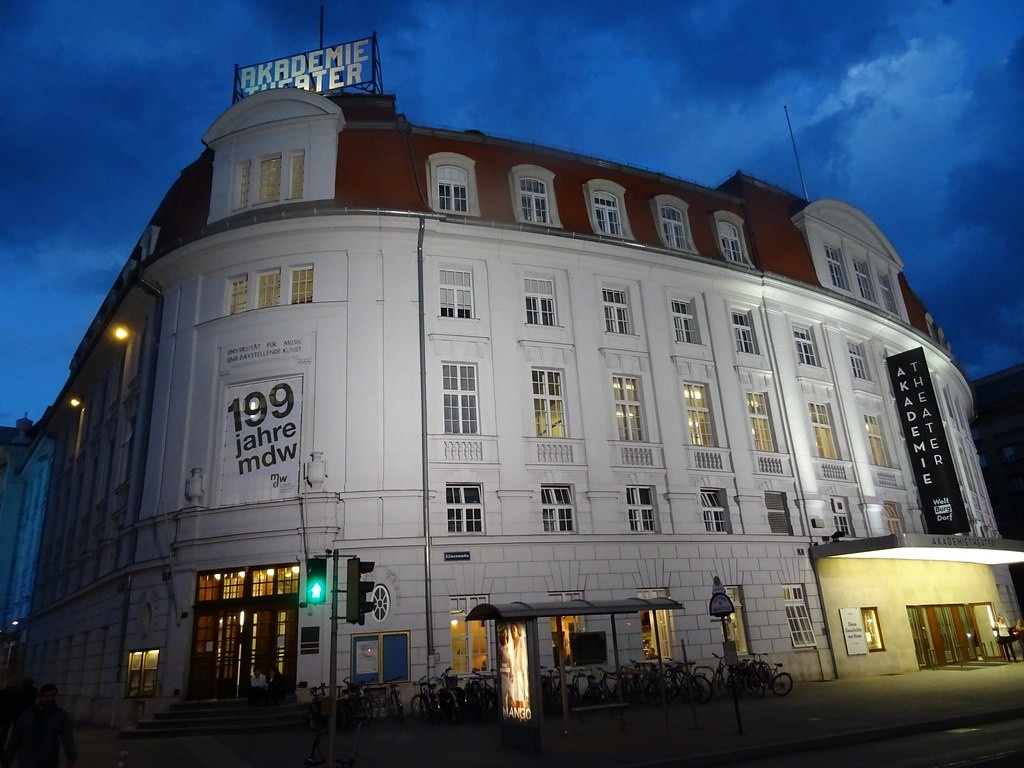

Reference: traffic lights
[345,559,376,625]
[307,557,327,605]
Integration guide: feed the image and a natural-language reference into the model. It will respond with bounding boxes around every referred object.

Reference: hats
[8,677,16,687]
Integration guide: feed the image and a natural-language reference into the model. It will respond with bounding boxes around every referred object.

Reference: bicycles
[304,649,792,727]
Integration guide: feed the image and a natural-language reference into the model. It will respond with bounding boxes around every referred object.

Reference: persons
[0,683,77,768]
[993,614,1018,662]
[0,676,38,764]
[251,668,266,697]
[1013,619,1024,662]
[262,667,287,707]
